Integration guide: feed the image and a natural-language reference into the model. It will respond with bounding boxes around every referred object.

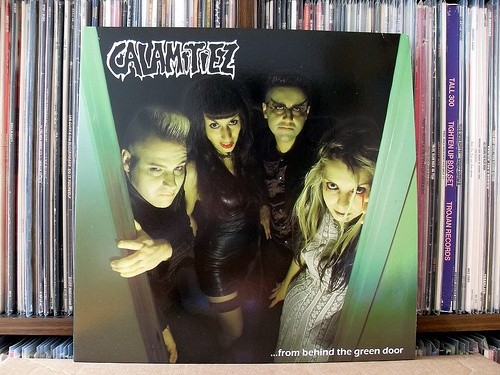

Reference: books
[1,1,499,363]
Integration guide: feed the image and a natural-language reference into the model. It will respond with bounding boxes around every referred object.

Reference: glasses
[265,102,306,115]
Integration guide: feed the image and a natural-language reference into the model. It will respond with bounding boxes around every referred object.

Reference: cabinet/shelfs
[0,1,500,339]
